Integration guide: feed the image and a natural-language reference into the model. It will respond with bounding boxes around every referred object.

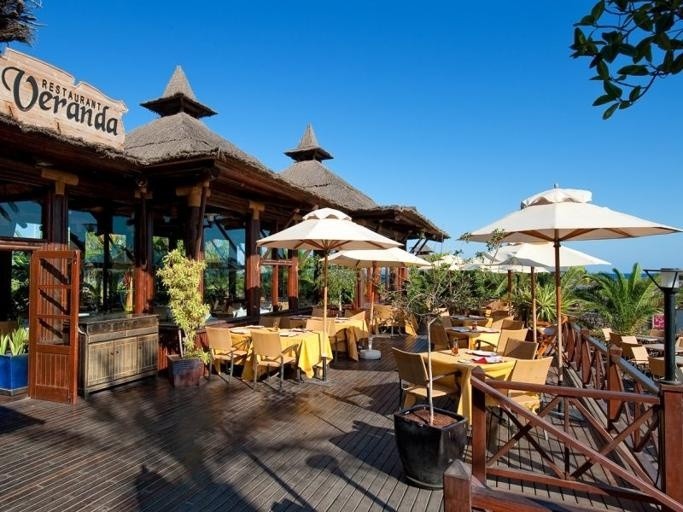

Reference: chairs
[204,304,419,394]
[603,326,682,386]
[391,305,560,444]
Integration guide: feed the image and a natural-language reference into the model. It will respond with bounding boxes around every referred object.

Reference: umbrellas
[320,246,431,319]
[455,184,683,412]
[418,253,465,295]
[448,266,549,319]
[257,208,404,336]
[462,241,612,343]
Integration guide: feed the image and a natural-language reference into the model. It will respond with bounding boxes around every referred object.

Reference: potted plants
[0,326,29,397]
[153,238,216,393]
[381,229,526,491]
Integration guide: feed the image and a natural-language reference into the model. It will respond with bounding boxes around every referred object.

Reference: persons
[123,256,154,313]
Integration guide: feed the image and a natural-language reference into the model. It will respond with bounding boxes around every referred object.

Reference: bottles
[453,338,458,354]
[273,322,279,332]
[473,320,477,329]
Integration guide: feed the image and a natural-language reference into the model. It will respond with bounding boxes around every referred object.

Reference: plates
[453,315,485,321]
[280,328,310,337]
[230,326,263,334]
[335,317,350,324]
[475,357,500,363]
[472,350,497,356]
